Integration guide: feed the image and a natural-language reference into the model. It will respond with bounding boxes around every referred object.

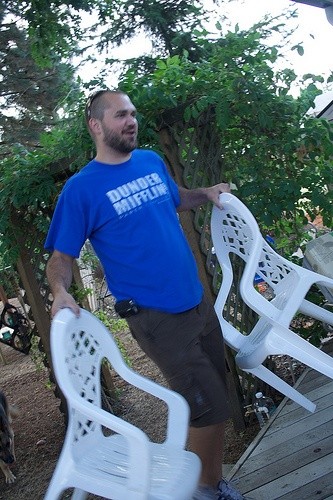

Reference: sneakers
[193,477,247,500]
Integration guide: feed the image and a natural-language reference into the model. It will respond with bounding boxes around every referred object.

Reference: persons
[44,91,248,500]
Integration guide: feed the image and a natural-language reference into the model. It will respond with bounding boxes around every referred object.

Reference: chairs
[42,306,202,499]
[209,192,333,413]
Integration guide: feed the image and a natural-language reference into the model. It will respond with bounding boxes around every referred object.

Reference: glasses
[86,91,109,118]
